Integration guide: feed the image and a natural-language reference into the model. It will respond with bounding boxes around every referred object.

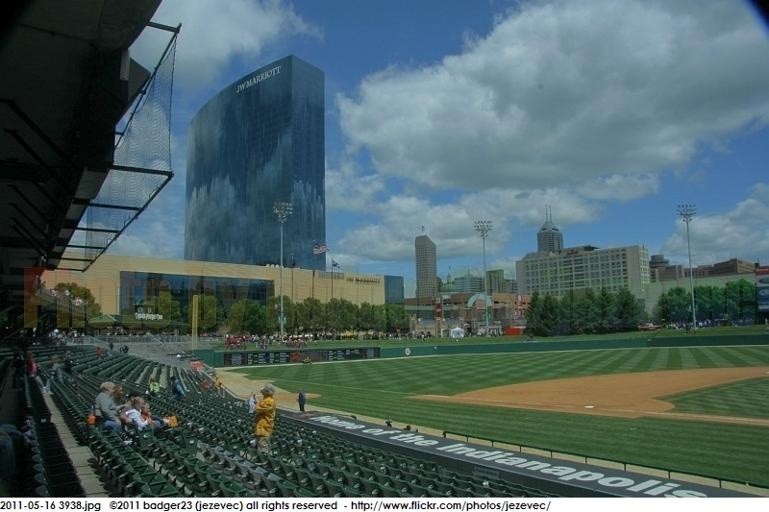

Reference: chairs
[0,399,558,496]
[1,345,223,400]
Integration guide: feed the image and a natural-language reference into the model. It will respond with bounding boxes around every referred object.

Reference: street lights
[676,204,696,327]
[475,221,493,337]
[273,200,293,340]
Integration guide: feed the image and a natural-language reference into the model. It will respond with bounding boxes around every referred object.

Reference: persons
[96,347,101,359]
[170,376,186,400]
[224,329,433,350]
[148,378,161,399]
[254,383,277,463]
[13,349,76,384]
[247,392,257,414]
[110,342,114,351]
[197,378,209,402]
[297,389,305,411]
[213,375,223,397]
[92,382,177,444]
